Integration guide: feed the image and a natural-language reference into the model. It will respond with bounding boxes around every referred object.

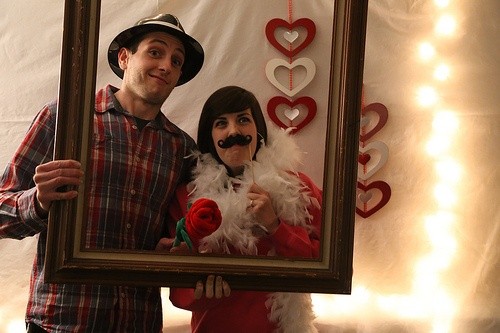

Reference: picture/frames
[43,0,368,295]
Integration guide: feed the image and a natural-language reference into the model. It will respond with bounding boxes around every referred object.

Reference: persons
[0,12,231,332]
[168,85,328,333]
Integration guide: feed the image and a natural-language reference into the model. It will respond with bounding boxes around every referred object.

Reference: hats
[108,12,205,87]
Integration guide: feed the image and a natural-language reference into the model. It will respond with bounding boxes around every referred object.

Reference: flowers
[172,198,223,253]
